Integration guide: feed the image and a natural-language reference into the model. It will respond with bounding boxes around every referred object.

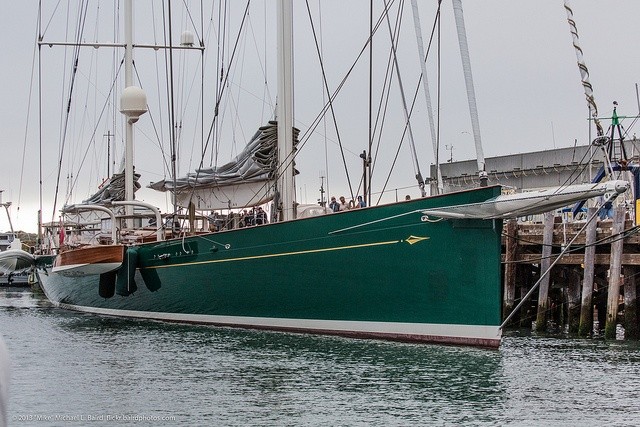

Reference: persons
[326,196,365,214]
[208,208,270,231]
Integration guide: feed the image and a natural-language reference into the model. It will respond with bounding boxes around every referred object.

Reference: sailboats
[35,0,631,349]
[0,190,35,275]
